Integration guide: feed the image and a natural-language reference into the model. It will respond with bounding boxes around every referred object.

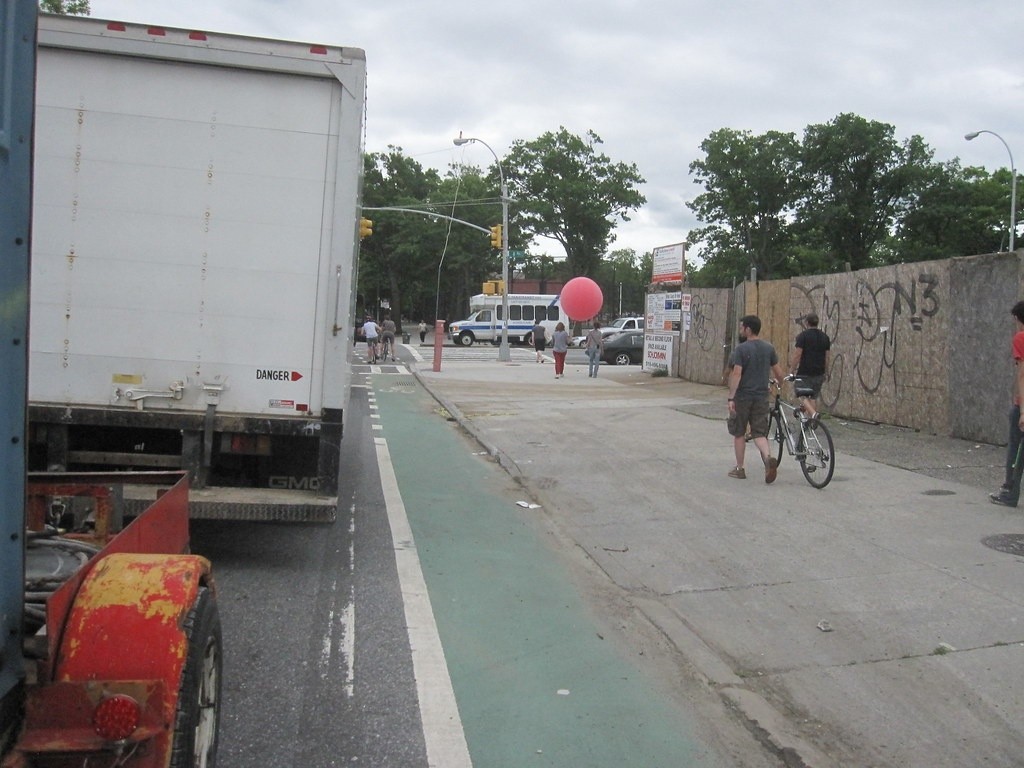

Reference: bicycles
[376,329,390,362]
[363,331,379,364]
[760,374,836,489]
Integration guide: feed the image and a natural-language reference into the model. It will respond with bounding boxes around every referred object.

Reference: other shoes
[989,486,1019,507]
[765,457,777,483]
[728,466,746,479]
[812,412,821,430]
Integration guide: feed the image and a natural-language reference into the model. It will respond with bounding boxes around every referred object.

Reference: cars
[571,336,587,349]
[584,331,644,366]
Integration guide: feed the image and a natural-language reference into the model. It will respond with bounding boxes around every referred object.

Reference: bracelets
[728,399,734,401]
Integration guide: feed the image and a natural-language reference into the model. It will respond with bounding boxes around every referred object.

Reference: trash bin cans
[402,331,411,344]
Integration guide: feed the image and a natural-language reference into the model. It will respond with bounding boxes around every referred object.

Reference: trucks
[22,13,366,526]
[448,294,569,346]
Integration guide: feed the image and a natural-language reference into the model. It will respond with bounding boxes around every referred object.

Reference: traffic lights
[491,224,501,247]
[360,217,372,239]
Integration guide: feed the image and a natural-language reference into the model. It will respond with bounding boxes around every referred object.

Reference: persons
[551,322,572,379]
[586,322,602,378]
[443,313,453,340]
[380,315,397,362]
[989,300,1024,508]
[789,312,830,430]
[727,314,783,484]
[361,315,382,364]
[532,320,548,363]
[418,320,427,342]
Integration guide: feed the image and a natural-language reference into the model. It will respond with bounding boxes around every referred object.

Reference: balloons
[560,276,603,321]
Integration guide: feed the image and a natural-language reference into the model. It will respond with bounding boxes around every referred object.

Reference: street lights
[453,138,512,362]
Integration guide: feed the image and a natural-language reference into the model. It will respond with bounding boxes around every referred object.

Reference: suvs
[588,316,644,338]
